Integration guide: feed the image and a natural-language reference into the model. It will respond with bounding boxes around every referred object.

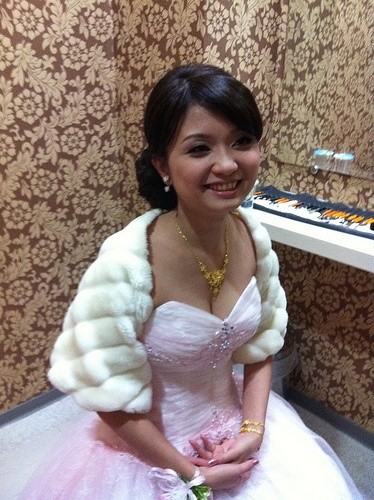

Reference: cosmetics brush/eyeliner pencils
[239,185,374,232]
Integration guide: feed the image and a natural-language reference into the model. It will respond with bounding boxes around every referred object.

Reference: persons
[0,63,362,500]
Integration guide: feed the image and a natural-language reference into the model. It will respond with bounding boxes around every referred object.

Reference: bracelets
[239,427,264,437]
[240,420,265,429]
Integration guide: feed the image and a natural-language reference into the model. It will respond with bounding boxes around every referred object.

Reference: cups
[334,153,355,174]
[240,185,255,208]
[315,150,331,169]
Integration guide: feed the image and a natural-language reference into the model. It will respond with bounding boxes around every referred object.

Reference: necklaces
[175,211,229,297]
